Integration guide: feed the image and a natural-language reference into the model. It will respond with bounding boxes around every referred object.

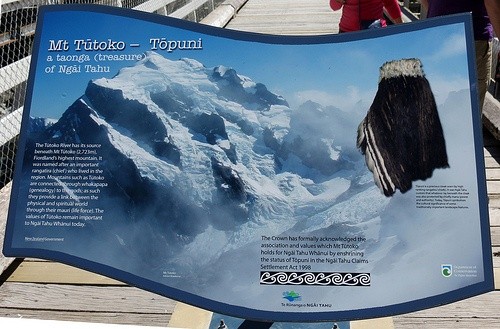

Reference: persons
[330,0,404,35]
[418,0,500,147]
